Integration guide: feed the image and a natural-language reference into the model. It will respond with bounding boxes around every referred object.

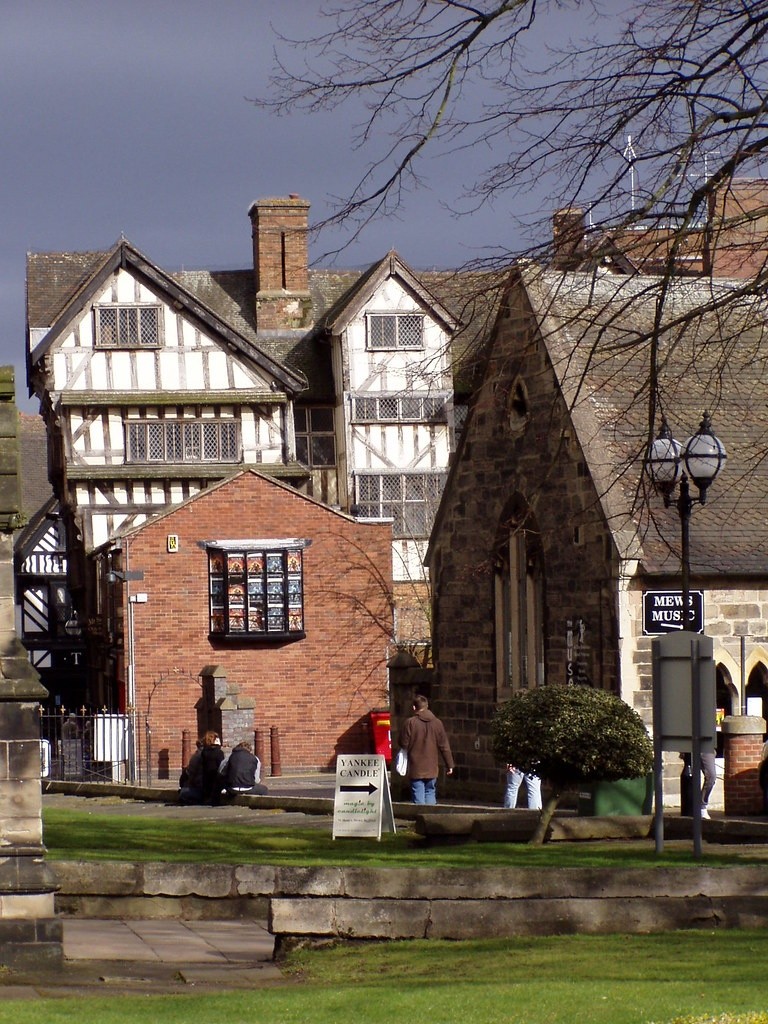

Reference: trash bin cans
[576,757,665,816]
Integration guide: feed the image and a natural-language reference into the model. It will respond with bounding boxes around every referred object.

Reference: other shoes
[701,809,711,819]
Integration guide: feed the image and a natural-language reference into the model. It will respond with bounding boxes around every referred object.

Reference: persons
[399,695,454,804]
[62,713,93,774]
[219,742,268,795]
[502,762,542,809]
[178,732,224,790]
[682,750,715,820]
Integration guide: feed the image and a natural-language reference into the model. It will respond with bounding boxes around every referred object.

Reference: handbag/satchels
[391,747,408,776]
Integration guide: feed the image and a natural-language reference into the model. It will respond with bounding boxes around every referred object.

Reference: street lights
[642,408,728,817]
